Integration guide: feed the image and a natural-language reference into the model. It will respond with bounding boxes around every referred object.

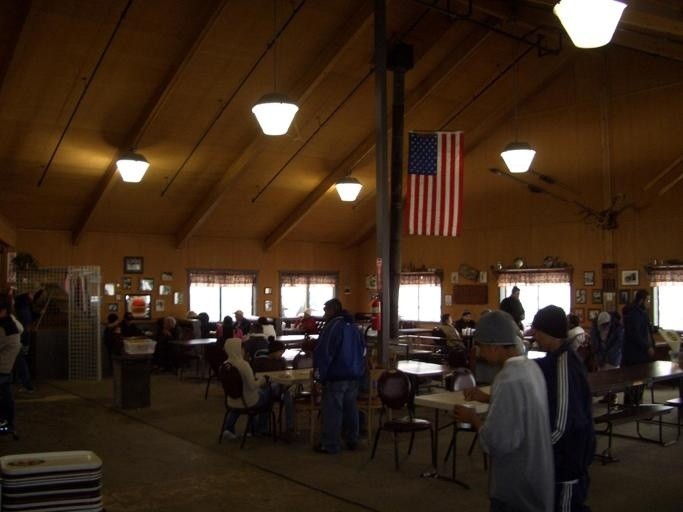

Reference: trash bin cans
[114,353,152,409]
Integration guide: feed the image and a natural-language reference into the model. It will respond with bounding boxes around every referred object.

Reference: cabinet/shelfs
[110,350,153,410]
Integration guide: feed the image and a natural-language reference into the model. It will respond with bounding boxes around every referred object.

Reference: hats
[474,309,521,347]
[512,284,521,295]
[107,314,118,324]
[268,341,286,354]
[531,304,570,340]
[123,311,135,321]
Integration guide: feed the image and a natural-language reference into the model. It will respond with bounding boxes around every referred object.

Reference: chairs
[166,317,682,492]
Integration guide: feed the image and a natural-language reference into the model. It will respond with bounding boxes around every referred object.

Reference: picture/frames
[573,263,639,323]
[103,256,183,319]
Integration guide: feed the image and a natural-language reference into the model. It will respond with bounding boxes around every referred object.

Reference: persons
[104,311,316,376]
[500,287,524,331]
[590,289,652,405]
[219,337,302,440]
[311,297,366,454]
[439,309,478,346]
[2,286,38,432]
[475,305,596,511]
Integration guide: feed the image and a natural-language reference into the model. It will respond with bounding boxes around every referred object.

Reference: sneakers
[312,442,338,455]
[598,394,612,405]
[608,394,618,405]
[342,440,357,451]
[17,386,34,394]
[222,429,238,441]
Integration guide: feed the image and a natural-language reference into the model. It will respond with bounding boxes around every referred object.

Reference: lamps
[252,32,299,136]
[114,137,149,185]
[501,77,536,176]
[552,0,629,51]
[335,168,363,204]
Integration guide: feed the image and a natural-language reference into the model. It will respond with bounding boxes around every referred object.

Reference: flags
[404,131,464,237]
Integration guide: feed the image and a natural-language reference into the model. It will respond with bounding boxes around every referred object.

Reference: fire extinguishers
[372,295,381,331]
